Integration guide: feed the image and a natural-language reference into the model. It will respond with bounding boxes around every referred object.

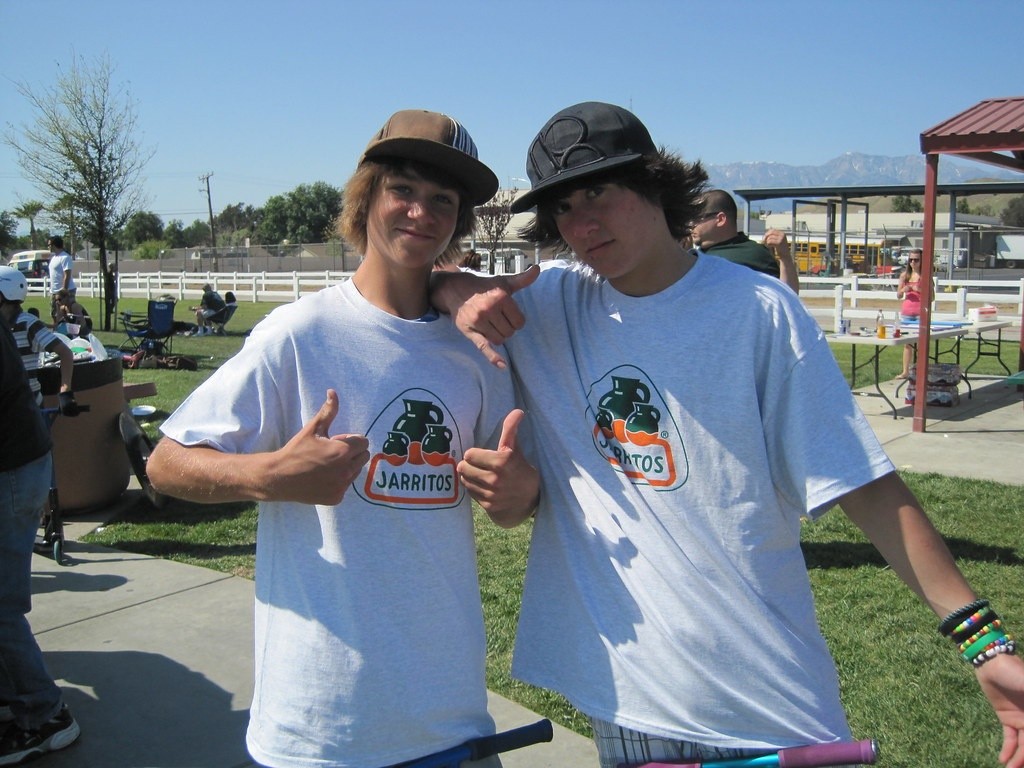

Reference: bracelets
[938,598,1015,664]
[192,306,195,311]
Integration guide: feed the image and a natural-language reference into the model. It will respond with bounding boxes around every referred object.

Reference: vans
[11,259,50,293]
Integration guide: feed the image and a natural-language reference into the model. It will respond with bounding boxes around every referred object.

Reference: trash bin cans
[38,348,130,515]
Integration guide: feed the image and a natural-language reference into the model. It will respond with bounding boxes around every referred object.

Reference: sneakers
[0,700,15,722]
[0,703,81,765]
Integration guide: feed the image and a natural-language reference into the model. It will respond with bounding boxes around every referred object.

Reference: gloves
[57,391,79,417]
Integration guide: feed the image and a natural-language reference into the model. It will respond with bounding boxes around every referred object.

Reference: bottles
[148,339,154,348]
[839,320,845,335]
[877,314,885,339]
[70,346,92,353]
[893,313,900,337]
[875,309,882,332]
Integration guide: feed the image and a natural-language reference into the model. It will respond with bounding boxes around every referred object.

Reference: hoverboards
[32,402,95,566]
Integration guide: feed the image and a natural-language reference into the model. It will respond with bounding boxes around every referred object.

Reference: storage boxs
[969,307,997,321]
[905,363,963,407]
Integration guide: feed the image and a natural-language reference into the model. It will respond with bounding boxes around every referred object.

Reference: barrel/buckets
[147,300,174,336]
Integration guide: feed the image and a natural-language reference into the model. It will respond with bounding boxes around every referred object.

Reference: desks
[824,326,971,420]
[935,316,1013,378]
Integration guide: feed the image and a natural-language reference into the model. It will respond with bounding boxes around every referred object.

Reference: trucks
[995,234,1024,269]
[6,250,52,272]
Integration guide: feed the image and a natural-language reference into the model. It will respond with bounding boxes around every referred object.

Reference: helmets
[1,266,28,304]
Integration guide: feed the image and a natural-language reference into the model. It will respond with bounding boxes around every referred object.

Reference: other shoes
[894,375,908,380]
[193,331,205,336]
[203,331,214,336]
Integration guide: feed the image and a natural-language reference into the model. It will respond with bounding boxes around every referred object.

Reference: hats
[357,109,500,206]
[510,101,662,215]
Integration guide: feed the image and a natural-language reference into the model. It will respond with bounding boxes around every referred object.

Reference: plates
[132,405,156,416]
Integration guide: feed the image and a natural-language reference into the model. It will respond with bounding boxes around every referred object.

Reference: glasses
[47,243,53,247]
[690,211,719,225]
[907,258,920,262]
[54,296,66,302]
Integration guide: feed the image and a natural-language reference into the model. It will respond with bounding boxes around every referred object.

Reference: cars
[810,253,862,278]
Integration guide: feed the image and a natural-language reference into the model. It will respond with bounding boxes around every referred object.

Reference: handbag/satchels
[122,349,198,372]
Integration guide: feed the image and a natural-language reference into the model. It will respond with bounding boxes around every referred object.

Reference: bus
[790,234,888,274]
[875,248,967,269]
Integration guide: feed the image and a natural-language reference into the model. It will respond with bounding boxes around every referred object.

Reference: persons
[28,290,93,340]
[108,263,120,314]
[895,248,936,379]
[0,325,80,768]
[0,266,74,544]
[209,292,238,334]
[48,236,78,332]
[192,283,225,337]
[429,101,1024,768]
[691,189,798,295]
[145,108,539,768]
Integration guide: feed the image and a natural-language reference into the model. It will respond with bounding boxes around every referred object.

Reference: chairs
[117,293,180,357]
[203,304,237,336]
